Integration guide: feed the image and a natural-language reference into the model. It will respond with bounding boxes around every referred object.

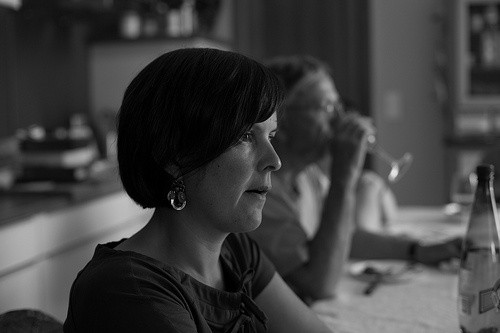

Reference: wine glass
[347,111,413,184]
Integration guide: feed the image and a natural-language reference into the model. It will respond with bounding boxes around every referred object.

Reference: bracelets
[408,239,418,263]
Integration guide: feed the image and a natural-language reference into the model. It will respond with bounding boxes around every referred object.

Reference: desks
[0,178,156,324]
[310,207,468,333]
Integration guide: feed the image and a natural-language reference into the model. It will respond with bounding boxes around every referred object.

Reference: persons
[64,48,333,333]
[245,55,465,306]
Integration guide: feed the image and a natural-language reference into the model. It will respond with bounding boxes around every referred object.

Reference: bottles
[457,164,500,333]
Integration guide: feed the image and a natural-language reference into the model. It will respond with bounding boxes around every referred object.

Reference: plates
[348,260,407,278]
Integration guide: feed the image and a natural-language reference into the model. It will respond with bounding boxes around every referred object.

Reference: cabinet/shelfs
[450,0,500,205]
